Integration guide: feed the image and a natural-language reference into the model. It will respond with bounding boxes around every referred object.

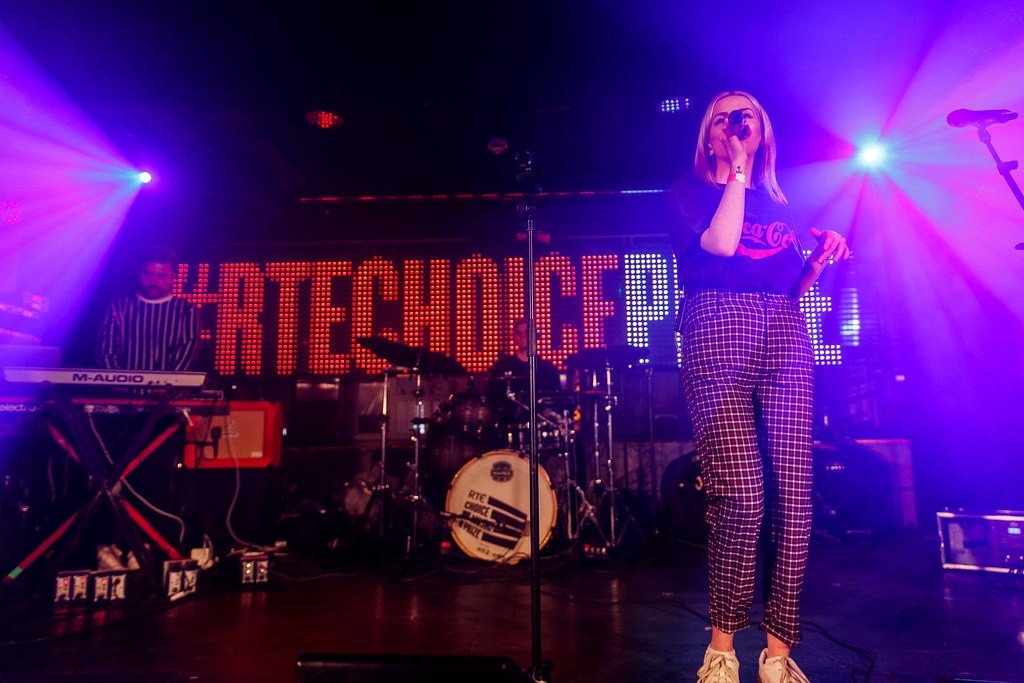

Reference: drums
[448,391,504,439]
[444,447,567,567]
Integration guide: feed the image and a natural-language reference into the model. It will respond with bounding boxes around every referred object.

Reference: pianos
[0,366,233,586]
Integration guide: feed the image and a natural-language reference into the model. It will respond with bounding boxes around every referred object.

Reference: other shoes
[128,551,139,570]
[98,544,125,570]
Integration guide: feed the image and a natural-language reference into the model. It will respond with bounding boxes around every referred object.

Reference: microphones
[211,427,222,457]
[728,110,747,139]
[947,108,1017,128]
[514,149,540,220]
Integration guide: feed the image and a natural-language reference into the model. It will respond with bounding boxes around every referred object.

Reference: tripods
[331,356,439,565]
[553,356,645,564]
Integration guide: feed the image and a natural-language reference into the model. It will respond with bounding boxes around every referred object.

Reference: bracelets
[726,173,746,185]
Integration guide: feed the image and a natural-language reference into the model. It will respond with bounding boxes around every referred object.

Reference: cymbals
[356,335,410,359]
[479,375,528,380]
[562,343,651,371]
[579,388,608,395]
[384,347,468,377]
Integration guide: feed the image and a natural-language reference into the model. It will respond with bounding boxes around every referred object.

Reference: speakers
[183,398,284,467]
[293,653,532,683]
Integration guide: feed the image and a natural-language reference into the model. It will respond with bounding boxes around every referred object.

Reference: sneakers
[757,647,811,683]
[696,645,739,683]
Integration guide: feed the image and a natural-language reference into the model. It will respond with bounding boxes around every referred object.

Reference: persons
[669,91,851,683]
[96,247,202,575]
[484,319,563,452]
